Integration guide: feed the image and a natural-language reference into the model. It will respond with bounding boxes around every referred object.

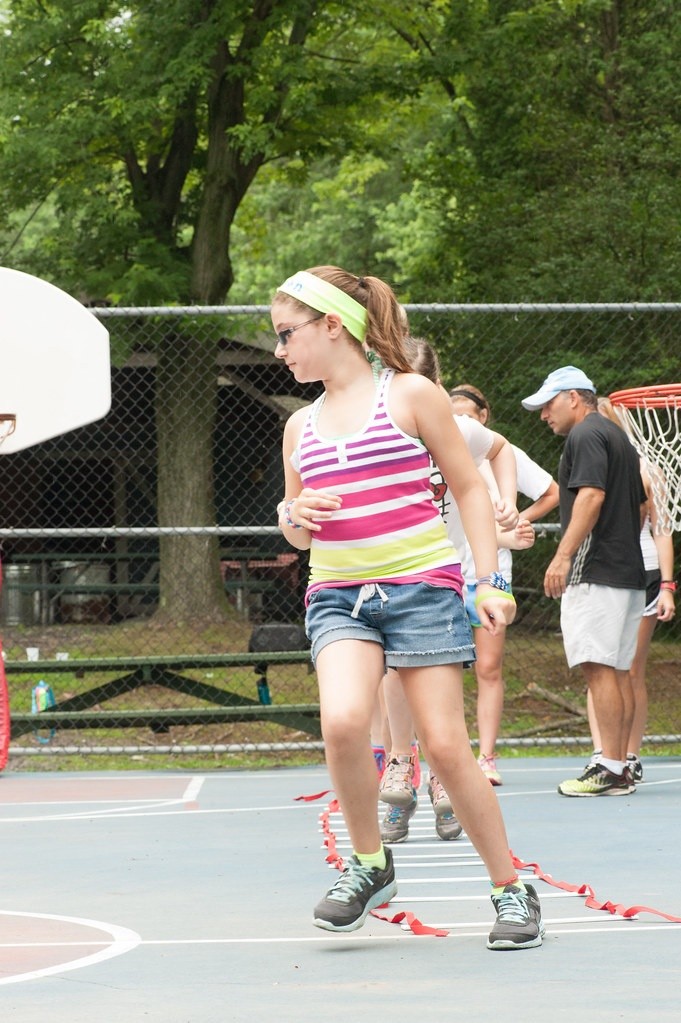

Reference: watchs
[660,580,678,592]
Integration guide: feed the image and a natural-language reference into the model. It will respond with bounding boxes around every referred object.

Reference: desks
[11,549,277,629]
[4,650,321,742]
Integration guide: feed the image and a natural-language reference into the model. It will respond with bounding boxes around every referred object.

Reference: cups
[25,647,39,662]
[56,652,69,661]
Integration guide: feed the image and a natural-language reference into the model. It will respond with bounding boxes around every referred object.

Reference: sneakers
[312,845,398,932]
[584,750,603,773]
[625,755,642,783]
[380,788,417,844]
[428,770,453,814]
[411,742,421,789]
[379,750,415,805]
[436,812,462,840]
[558,763,636,796]
[477,752,502,785]
[371,746,386,783]
[487,883,546,949]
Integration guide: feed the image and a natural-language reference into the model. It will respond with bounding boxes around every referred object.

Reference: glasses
[277,314,325,345]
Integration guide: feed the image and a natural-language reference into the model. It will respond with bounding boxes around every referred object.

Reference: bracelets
[478,573,507,590]
[286,498,303,529]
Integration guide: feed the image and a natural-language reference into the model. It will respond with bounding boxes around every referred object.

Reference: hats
[521,365,596,410]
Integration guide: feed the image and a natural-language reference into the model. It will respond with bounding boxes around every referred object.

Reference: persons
[369,306,519,843]
[584,397,678,783]
[522,366,648,797]
[449,384,560,785]
[271,265,546,950]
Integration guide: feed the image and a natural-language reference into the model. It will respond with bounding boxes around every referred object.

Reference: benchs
[10,703,321,725]
[5,579,274,605]
